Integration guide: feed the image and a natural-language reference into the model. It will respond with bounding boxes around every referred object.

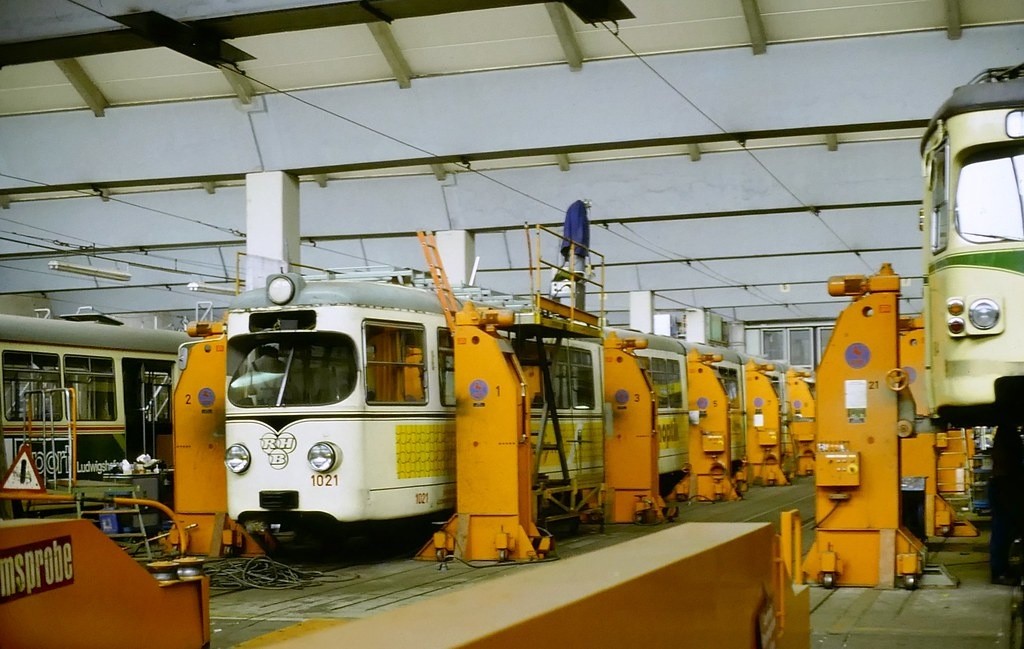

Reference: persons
[245,345,290,402]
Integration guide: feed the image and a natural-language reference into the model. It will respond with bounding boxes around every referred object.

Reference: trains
[224,271,818,544]
[920,55,1024,430]
[0,314,202,518]
